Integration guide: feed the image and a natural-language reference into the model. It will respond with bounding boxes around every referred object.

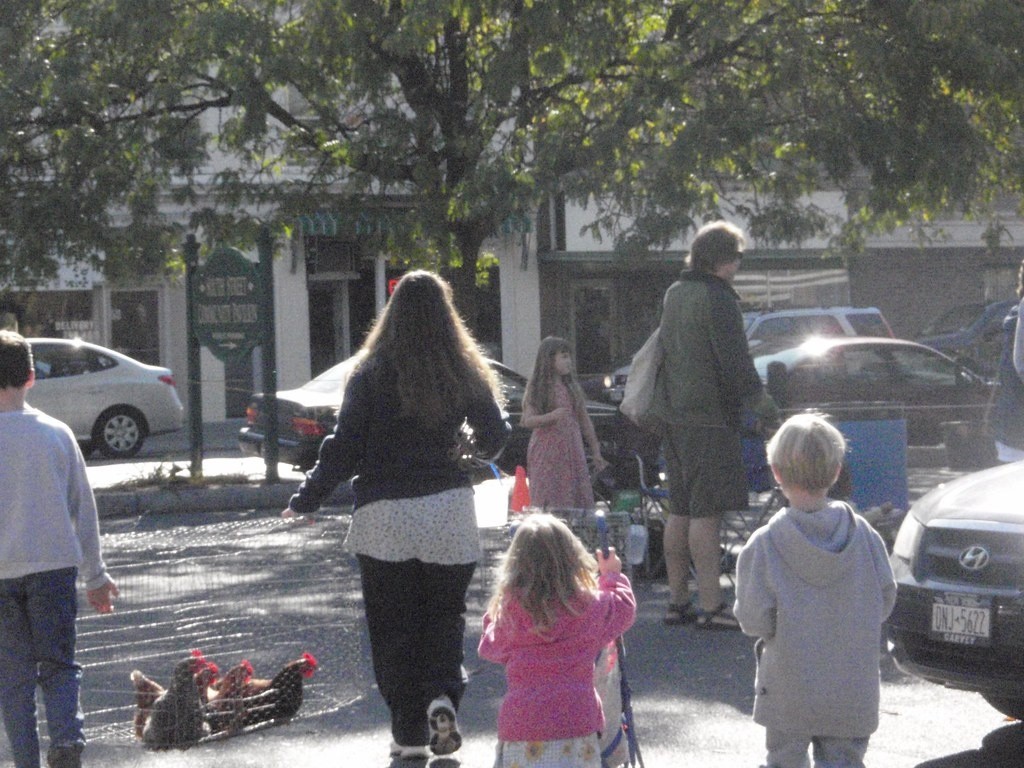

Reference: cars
[25,337,183,458]
[237,350,617,478]
[880,458,1024,720]
[602,298,1023,447]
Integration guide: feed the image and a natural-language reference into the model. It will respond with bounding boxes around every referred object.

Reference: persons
[280,269,513,759]
[651,220,783,631]
[731,412,898,767]
[994,260,1024,466]
[521,334,605,517]
[476,513,637,768]
[0,331,121,768]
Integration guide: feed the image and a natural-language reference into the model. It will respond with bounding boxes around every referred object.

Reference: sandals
[693,603,741,632]
[663,601,700,625]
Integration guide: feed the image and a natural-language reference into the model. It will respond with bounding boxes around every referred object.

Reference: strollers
[509,509,645,768]
[741,419,855,547]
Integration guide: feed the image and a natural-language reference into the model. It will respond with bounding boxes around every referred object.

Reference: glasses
[736,251,742,259]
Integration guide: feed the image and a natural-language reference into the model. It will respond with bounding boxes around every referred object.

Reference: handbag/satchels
[617,328,667,437]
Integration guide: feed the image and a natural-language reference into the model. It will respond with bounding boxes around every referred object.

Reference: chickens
[130,650,318,752]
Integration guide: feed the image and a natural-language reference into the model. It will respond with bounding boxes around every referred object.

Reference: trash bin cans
[778,399,908,511]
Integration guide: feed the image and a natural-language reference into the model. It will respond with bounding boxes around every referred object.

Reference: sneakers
[427,695,462,755]
[390,741,428,760]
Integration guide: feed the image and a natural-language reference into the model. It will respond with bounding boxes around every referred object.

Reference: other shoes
[48,749,81,768]
